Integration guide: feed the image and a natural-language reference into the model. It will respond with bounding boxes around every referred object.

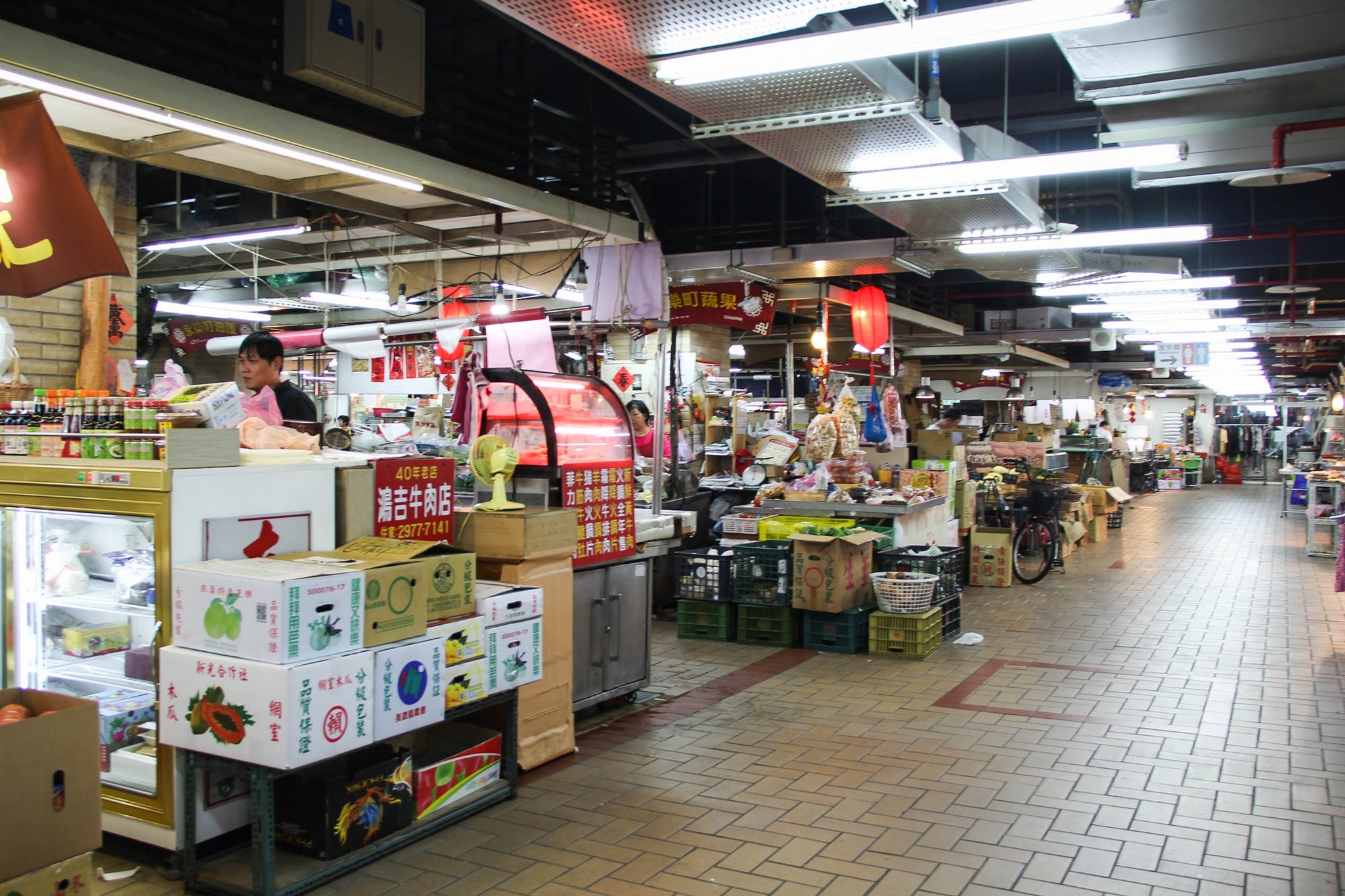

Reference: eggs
[826,454,865,484]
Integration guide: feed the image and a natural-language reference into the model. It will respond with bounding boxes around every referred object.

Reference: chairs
[1182,458,1203,490]
[1137,456,1162,493]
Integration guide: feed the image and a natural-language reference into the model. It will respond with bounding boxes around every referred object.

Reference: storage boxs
[669,418,1132,665]
[0,534,548,896]
[1013,307,1076,329]
[166,381,246,428]
[1158,479,1182,491]
[984,309,1014,330]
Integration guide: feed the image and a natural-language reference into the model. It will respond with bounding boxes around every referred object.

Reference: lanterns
[851,282,889,355]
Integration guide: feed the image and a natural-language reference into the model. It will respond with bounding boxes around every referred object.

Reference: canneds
[122,399,170,434]
[79,437,159,460]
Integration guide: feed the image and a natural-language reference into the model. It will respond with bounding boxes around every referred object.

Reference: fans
[469,434,527,513]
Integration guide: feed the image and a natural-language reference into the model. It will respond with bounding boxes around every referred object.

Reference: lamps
[889,253,936,279]
[915,376,935,399]
[842,141,1191,194]
[953,222,1214,256]
[1004,377,1025,399]
[140,214,313,252]
[0,61,424,193]
[647,0,1147,87]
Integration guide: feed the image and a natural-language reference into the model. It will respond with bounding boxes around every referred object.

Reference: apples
[0,704,57,726]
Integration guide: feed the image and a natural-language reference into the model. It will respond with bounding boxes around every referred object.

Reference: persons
[925,409,961,432]
[1095,421,1113,444]
[338,415,352,431]
[625,401,672,458]
[237,333,319,424]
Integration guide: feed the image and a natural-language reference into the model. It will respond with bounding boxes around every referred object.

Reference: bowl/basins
[826,450,868,483]
[136,722,157,746]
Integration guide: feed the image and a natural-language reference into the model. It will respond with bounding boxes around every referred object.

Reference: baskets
[868,571,939,613]
[720,512,779,534]
[0,356,34,404]
[758,516,856,541]
[1106,512,1123,528]
[845,524,895,550]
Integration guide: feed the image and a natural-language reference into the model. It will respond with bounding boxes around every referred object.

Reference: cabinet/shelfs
[1302,481,1343,558]
[1278,465,1308,520]
[703,392,748,479]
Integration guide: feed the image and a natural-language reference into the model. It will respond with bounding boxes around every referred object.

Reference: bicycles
[1002,457,1072,586]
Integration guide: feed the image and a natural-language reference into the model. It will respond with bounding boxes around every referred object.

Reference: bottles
[879,462,900,488]
[0,389,151,459]
[755,420,761,432]
[747,421,752,434]
